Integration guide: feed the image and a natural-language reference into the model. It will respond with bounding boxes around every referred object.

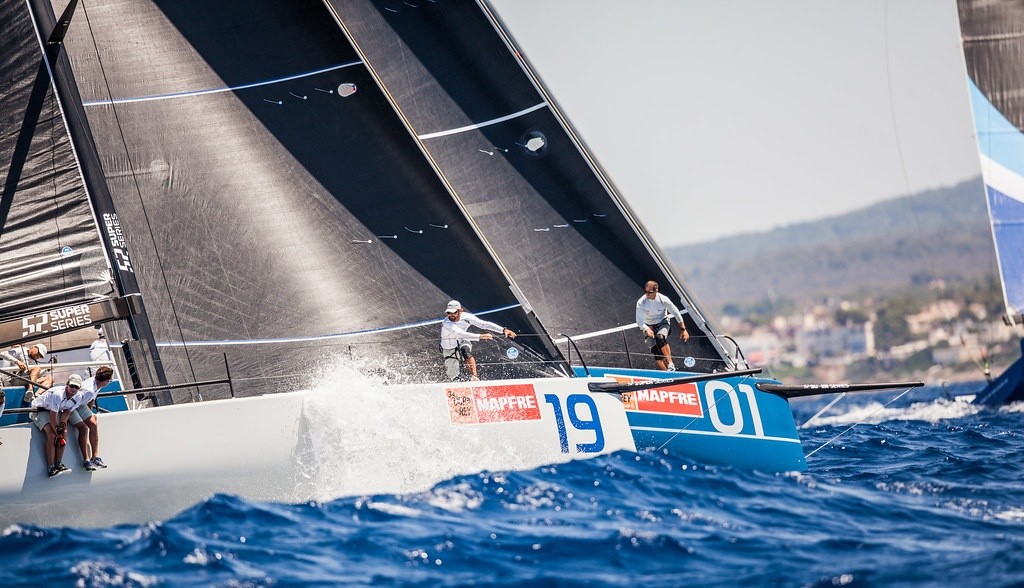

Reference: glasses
[643,289,655,294]
[69,384,81,389]
[447,311,457,314]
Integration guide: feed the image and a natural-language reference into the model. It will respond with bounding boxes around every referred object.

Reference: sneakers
[48,466,65,478]
[84,460,96,471]
[57,464,72,475]
[90,457,107,468]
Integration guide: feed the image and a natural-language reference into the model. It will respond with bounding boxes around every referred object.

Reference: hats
[68,374,82,387]
[98,327,105,336]
[445,300,461,313]
[36,344,47,359]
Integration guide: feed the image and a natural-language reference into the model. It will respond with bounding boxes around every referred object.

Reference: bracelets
[502,327,507,333]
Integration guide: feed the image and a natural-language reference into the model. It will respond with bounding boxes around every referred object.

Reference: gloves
[55,437,66,447]
[55,423,66,434]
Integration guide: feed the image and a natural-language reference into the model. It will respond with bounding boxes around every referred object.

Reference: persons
[440,300,517,382]
[635,281,690,372]
[0,328,117,479]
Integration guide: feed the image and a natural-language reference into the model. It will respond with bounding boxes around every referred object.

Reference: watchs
[681,328,686,331]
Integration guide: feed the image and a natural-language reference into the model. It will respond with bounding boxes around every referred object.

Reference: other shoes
[667,365,675,371]
[24,391,32,402]
[471,375,479,380]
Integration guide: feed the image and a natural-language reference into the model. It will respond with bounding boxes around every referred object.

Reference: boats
[1,0,925,553]
[953,0,1024,409]
